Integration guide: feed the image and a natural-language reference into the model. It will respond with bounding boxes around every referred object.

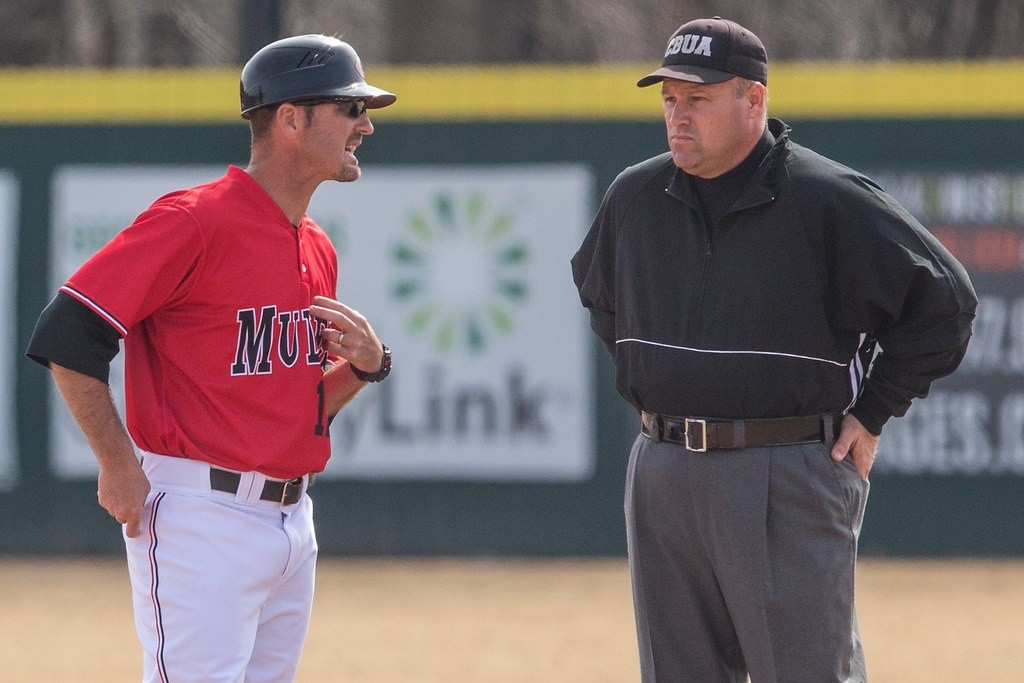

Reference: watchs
[350,343,393,383]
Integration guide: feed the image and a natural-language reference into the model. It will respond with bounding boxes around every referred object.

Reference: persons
[572,19,978,683]
[25,33,399,683]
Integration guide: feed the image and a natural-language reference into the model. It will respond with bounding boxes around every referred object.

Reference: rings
[338,332,344,344]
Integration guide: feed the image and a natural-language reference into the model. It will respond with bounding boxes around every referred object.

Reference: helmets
[240,34,397,120]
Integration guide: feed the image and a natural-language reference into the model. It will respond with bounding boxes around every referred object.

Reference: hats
[637,17,768,88]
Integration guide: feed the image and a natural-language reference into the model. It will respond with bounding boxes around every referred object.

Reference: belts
[640,409,844,452]
[139,456,304,507]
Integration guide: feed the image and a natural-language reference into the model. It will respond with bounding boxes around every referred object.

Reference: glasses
[267,96,367,118]
